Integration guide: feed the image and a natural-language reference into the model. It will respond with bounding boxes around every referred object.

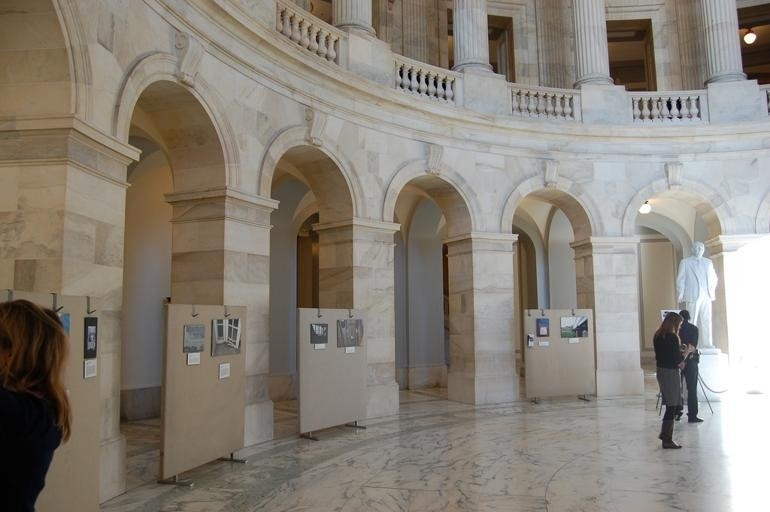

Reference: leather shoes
[686,416,705,423]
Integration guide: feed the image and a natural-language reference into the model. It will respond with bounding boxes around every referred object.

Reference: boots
[657,417,684,449]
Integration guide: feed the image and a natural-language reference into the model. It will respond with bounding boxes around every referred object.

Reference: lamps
[744,26,757,44]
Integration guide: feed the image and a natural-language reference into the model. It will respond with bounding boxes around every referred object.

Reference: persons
[675,310,704,423]
[653,312,697,449]
[674,240,719,350]
[1,299,74,511]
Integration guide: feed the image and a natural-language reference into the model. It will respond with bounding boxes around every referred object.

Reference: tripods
[653,358,715,416]
